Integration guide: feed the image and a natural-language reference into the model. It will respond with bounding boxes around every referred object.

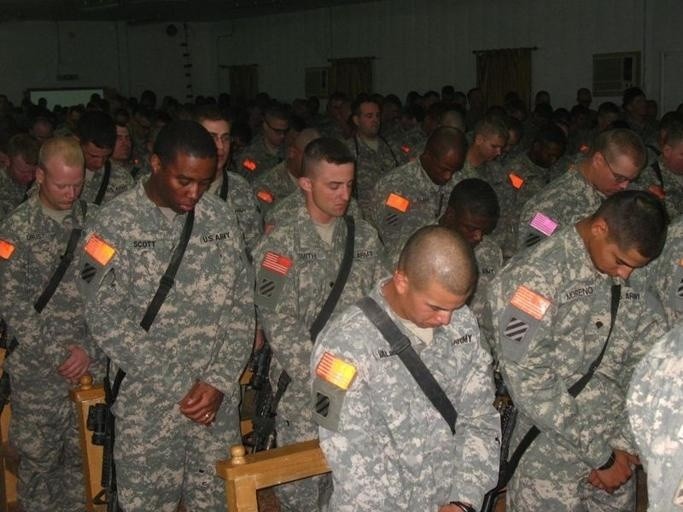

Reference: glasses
[214,131,233,147]
[601,154,633,184]
[265,119,289,134]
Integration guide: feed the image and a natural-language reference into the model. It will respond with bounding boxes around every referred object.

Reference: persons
[554,108,570,126]
[505,91,519,106]
[248,127,322,216]
[507,119,523,145]
[466,87,484,131]
[526,103,553,144]
[576,88,592,108]
[447,117,513,198]
[345,96,407,200]
[238,111,298,185]
[423,91,440,109]
[510,100,528,122]
[571,105,590,130]
[431,178,512,413]
[255,137,395,448]
[0,137,108,512]
[189,105,265,248]
[486,105,508,117]
[576,101,621,156]
[80,118,257,512]
[111,121,131,168]
[535,90,550,110]
[28,116,54,144]
[618,87,655,135]
[647,101,659,124]
[442,85,455,102]
[624,322,683,511]
[309,226,502,511]
[452,91,468,111]
[0,135,39,220]
[511,124,575,215]
[400,104,465,166]
[368,125,469,268]
[488,188,668,511]
[320,93,352,140]
[516,127,648,253]
[635,123,683,221]
[75,110,135,207]
[381,93,403,112]
